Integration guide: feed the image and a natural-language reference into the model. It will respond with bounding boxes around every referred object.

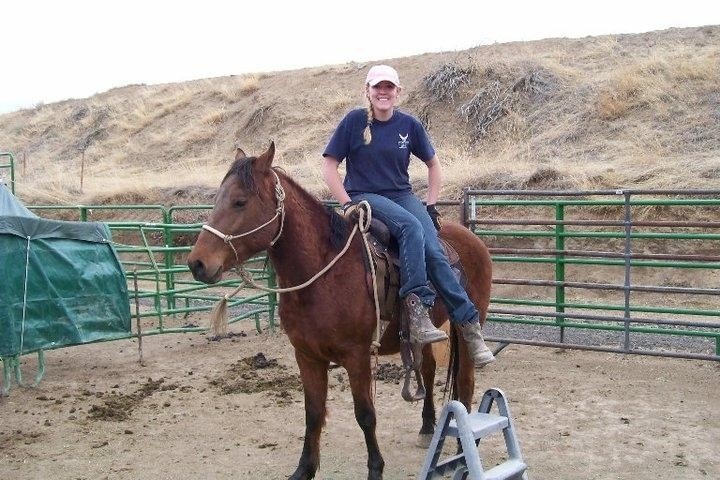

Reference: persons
[320,62,497,368]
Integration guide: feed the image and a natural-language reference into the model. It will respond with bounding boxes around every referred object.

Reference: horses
[186,139,494,480]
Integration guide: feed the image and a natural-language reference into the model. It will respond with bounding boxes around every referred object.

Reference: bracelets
[343,201,356,215]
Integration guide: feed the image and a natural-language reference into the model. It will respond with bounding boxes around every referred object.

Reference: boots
[403,292,449,344]
[458,322,496,369]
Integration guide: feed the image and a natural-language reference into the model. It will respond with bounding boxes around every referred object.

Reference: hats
[365,64,401,88]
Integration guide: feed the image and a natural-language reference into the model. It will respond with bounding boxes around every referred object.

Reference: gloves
[427,204,443,232]
[342,201,366,223]
